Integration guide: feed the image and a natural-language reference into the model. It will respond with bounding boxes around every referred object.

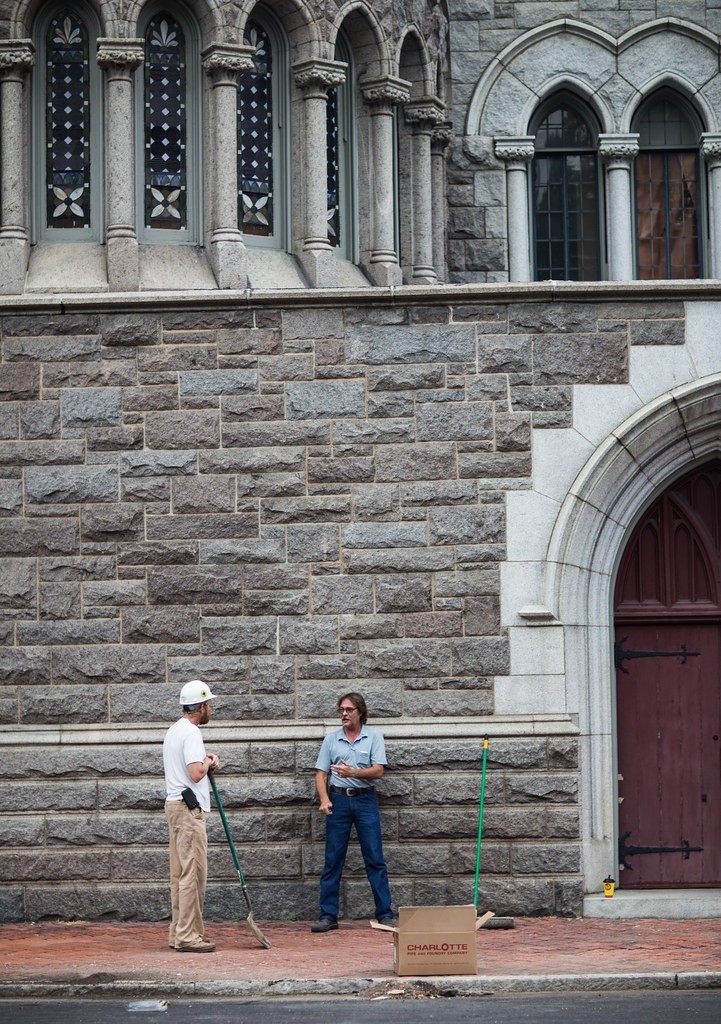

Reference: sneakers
[378,917,394,932]
[170,936,216,953]
[311,918,338,932]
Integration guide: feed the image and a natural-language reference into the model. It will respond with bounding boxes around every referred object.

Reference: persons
[163,680,220,952]
[311,693,395,931]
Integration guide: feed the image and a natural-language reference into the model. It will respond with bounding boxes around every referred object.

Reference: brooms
[469,733,517,929]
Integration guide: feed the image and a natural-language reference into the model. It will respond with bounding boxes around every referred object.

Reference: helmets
[179,680,218,705]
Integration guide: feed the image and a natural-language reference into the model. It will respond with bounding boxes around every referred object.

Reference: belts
[329,785,374,796]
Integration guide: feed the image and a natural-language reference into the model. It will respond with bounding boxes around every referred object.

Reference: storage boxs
[369,905,495,975]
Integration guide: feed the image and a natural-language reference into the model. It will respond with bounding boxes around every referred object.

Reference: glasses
[337,708,358,713]
